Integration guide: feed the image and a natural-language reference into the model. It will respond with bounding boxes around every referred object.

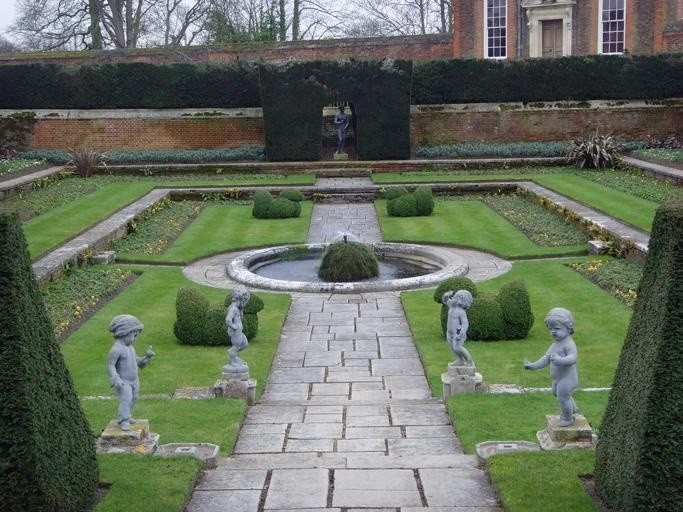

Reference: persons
[225,286,250,367]
[334,105,349,154]
[442,289,475,367]
[525,307,579,427]
[107,315,151,431]
